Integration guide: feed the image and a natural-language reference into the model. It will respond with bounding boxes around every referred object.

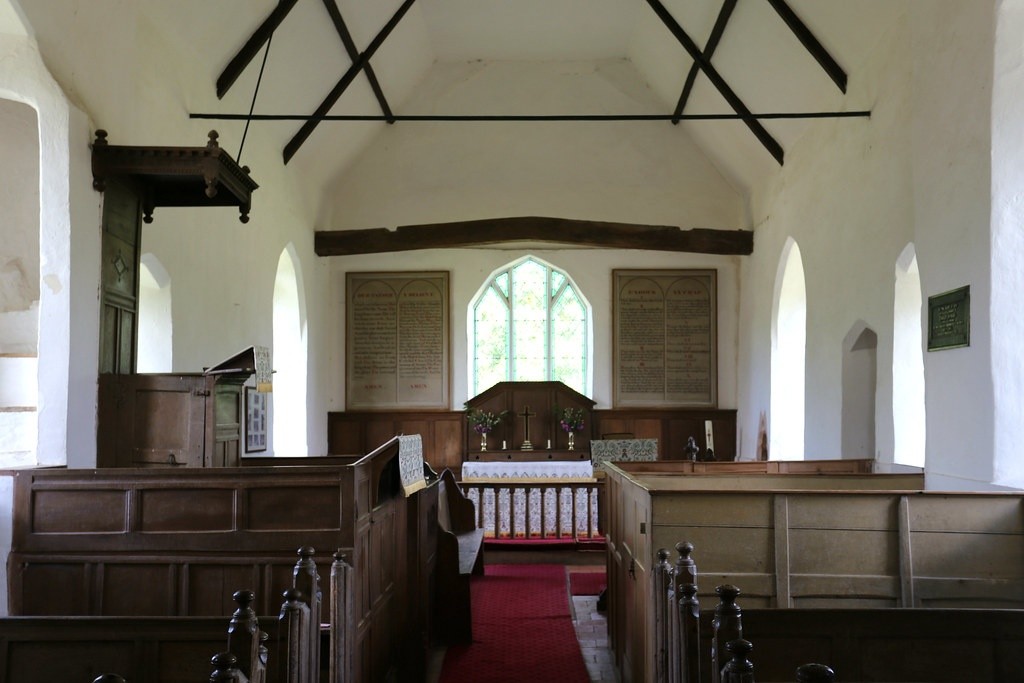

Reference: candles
[548,440,550,448]
[503,441,506,448]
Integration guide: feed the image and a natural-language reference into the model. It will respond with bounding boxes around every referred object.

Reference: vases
[568,431,574,451]
[481,433,487,451]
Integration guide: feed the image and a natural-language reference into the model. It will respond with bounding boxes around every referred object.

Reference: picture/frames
[245,386,268,453]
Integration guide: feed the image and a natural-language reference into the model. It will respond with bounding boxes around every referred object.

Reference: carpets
[439,563,592,683]
[571,573,607,595]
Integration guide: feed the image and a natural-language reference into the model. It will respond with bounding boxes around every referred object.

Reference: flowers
[553,403,584,431]
[463,401,508,432]
